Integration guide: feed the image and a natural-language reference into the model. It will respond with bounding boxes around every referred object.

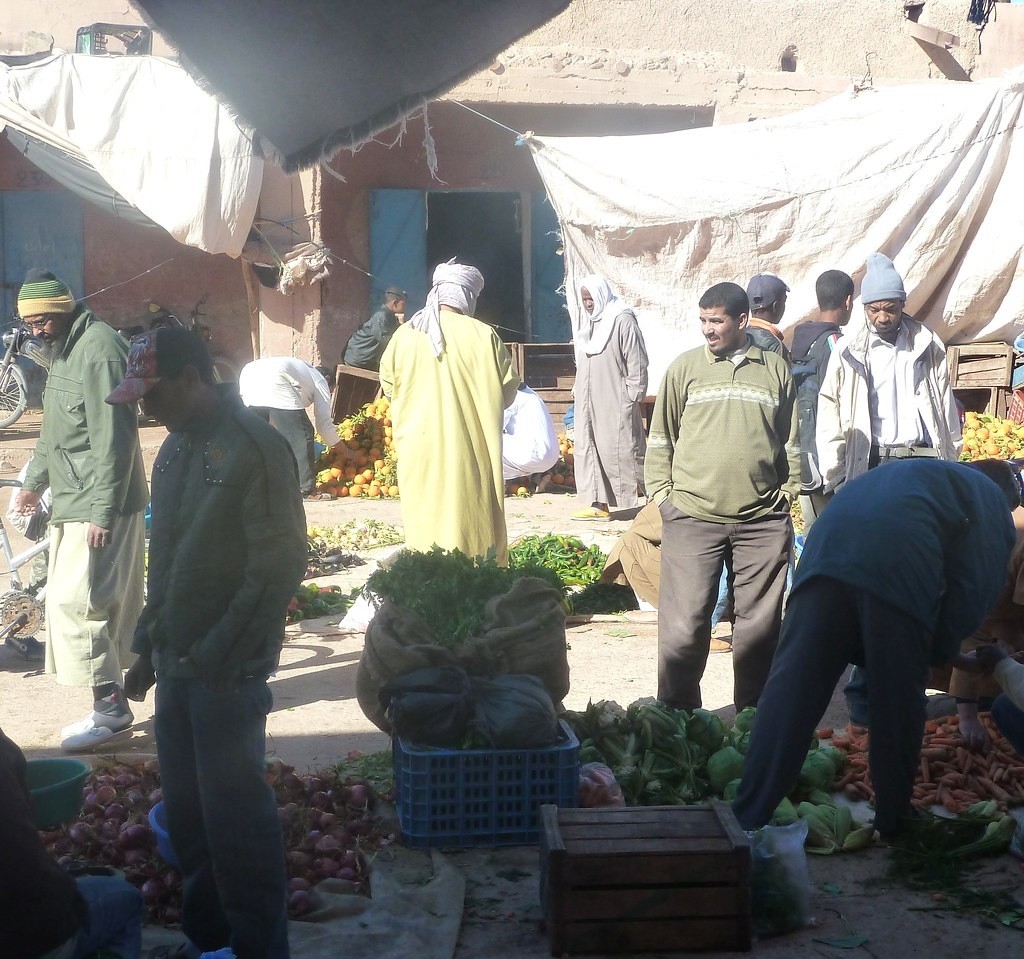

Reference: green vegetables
[333,749,394,795]
[362,542,574,647]
[864,793,1017,890]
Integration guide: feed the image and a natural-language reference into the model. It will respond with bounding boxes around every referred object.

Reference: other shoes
[708,636,732,653]
[847,721,869,744]
[879,803,920,842]
[624,609,658,624]
[535,474,550,493]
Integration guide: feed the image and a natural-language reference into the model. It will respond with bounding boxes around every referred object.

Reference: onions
[38,758,370,925]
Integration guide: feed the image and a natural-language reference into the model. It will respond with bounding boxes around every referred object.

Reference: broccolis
[565,696,694,800]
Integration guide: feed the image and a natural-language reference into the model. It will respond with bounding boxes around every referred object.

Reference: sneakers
[570,502,610,521]
[4,633,45,662]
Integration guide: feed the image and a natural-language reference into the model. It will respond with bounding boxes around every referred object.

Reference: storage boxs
[325,341,578,437]
[539,798,752,957]
[393,716,580,850]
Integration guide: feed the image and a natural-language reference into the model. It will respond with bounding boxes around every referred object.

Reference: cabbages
[686,706,845,800]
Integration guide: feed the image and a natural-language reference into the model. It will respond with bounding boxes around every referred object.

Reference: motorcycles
[0,292,241,429]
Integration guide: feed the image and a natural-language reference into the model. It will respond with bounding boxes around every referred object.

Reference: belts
[870,444,939,457]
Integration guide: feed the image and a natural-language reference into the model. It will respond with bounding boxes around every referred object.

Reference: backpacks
[790,330,844,495]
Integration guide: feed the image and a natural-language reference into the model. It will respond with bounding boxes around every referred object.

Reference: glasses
[25,314,51,329]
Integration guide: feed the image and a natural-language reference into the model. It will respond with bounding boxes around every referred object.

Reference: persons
[104,326,308,959]
[15,268,150,754]
[731,459,1021,844]
[239,252,1024,762]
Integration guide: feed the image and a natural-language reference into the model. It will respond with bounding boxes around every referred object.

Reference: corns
[775,790,874,854]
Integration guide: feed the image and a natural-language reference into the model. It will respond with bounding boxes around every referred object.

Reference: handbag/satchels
[743,821,810,936]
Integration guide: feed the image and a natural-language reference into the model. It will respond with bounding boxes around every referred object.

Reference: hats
[747,275,790,310]
[105,327,209,406]
[860,253,906,305]
[17,268,74,318]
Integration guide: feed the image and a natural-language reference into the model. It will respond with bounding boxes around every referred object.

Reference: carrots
[579,766,623,807]
[819,712,1024,812]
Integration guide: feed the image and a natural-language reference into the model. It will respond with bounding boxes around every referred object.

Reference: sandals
[302,492,336,502]
[149,943,200,959]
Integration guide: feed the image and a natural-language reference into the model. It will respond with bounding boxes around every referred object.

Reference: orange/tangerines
[316,397,398,497]
[506,433,576,493]
[962,412,1024,462]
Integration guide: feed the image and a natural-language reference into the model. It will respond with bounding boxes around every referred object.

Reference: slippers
[59,710,134,753]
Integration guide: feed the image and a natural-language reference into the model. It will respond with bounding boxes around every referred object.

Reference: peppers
[506,532,608,586]
[288,583,341,620]
